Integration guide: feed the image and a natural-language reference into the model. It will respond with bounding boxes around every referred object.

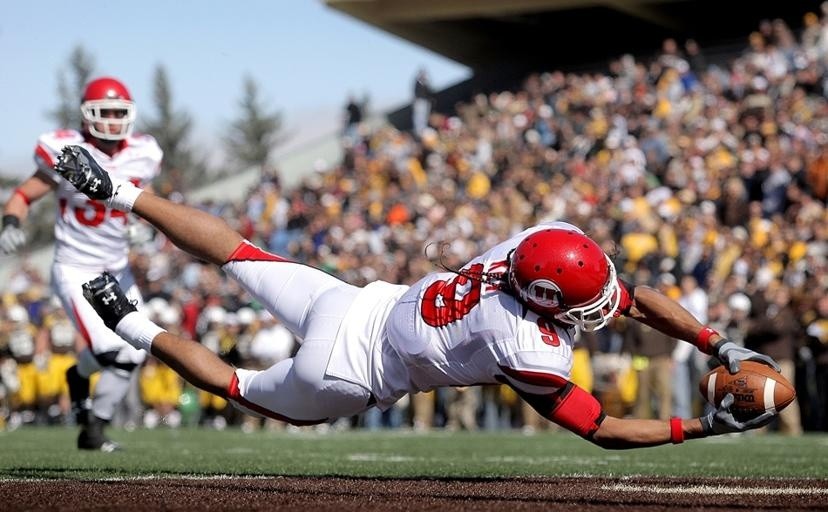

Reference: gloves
[714,336,781,376]
[0,221,30,257]
[697,390,779,435]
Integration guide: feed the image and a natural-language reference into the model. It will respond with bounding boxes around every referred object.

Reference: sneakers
[81,271,129,322]
[51,141,115,201]
[75,429,128,455]
[64,361,94,431]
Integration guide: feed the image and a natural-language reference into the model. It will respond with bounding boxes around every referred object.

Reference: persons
[0,76,166,454]
[1,1,828,438]
[47,145,784,452]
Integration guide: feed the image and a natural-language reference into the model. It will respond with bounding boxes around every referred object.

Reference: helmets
[504,224,619,333]
[78,76,137,145]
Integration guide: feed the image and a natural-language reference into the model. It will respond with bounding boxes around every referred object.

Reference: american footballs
[699,361,797,421]
